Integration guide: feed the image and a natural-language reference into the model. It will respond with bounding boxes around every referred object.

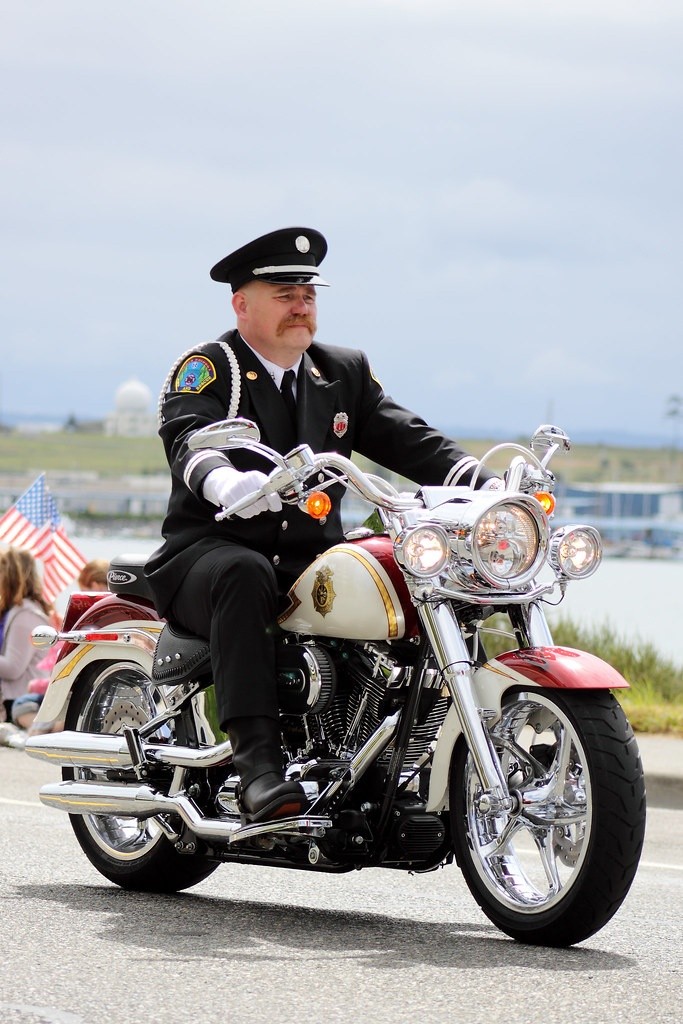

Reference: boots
[224,715,307,828]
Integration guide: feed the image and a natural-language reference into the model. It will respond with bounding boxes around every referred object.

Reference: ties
[279,370,297,439]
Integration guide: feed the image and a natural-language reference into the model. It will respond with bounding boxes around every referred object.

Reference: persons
[143,226,507,827]
[0,550,110,732]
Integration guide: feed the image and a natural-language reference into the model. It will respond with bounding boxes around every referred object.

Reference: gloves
[216,470,282,518]
[481,477,506,491]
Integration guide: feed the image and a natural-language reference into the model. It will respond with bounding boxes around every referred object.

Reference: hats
[209,227,330,294]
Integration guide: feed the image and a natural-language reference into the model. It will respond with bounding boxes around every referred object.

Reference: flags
[0,476,89,606]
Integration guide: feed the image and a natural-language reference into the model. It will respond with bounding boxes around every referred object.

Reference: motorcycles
[25,417,647,946]
